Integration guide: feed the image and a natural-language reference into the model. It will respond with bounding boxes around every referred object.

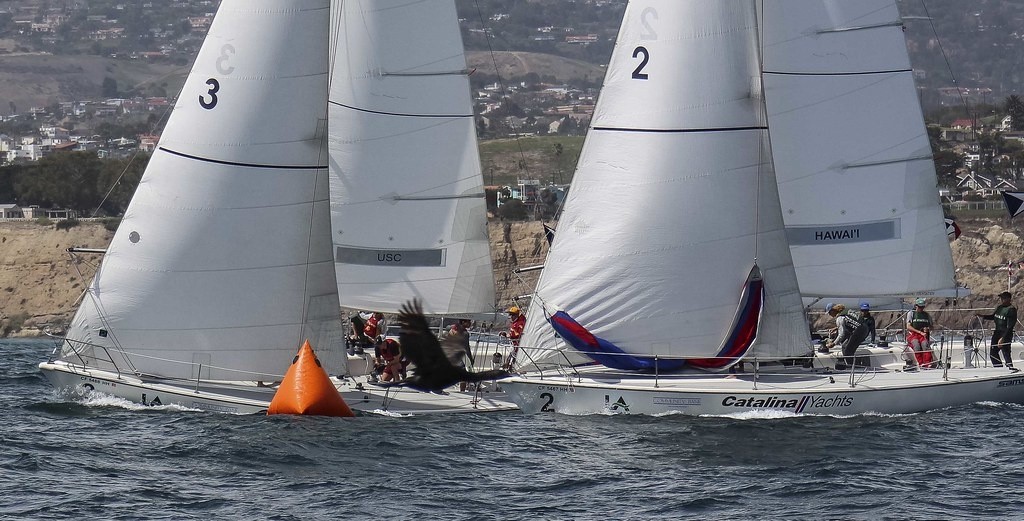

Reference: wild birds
[367,296,514,393]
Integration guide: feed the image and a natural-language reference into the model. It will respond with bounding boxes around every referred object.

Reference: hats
[915,299,925,307]
[373,333,386,346]
[505,306,519,313]
[998,292,1012,300]
[825,303,836,314]
[860,303,870,309]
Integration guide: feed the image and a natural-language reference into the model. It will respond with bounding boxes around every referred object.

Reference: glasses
[509,313,517,316]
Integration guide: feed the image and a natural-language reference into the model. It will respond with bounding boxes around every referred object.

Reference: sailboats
[37,0,1024,417]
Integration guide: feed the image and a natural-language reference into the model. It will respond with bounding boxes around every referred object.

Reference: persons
[975,291,1017,368]
[375,334,411,382]
[907,298,934,368]
[858,303,876,344]
[498,306,526,364]
[351,310,386,340]
[825,304,869,364]
[447,319,474,391]
